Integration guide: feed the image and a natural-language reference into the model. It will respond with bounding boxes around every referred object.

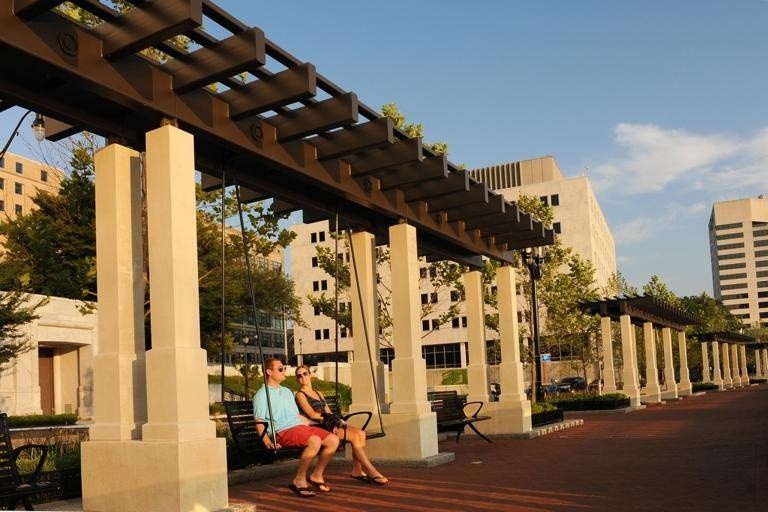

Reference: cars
[523,376,604,397]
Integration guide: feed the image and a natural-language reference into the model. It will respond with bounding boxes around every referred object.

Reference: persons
[250,358,339,498]
[294,365,389,485]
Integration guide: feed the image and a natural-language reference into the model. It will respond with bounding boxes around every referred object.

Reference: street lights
[240,331,249,400]
[254,334,258,363]
[0,110,44,161]
[299,338,303,355]
[521,212,552,402]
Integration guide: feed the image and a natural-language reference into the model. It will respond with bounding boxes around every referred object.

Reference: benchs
[221,396,386,461]
[427,390,494,452]
[0,413,61,510]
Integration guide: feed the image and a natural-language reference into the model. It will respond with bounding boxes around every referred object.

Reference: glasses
[268,366,288,373]
[296,371,309,378]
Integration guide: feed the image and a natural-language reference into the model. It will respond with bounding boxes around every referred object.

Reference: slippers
[307,478,332,492]
[289,484,315,497]
[350,474,390,485]
